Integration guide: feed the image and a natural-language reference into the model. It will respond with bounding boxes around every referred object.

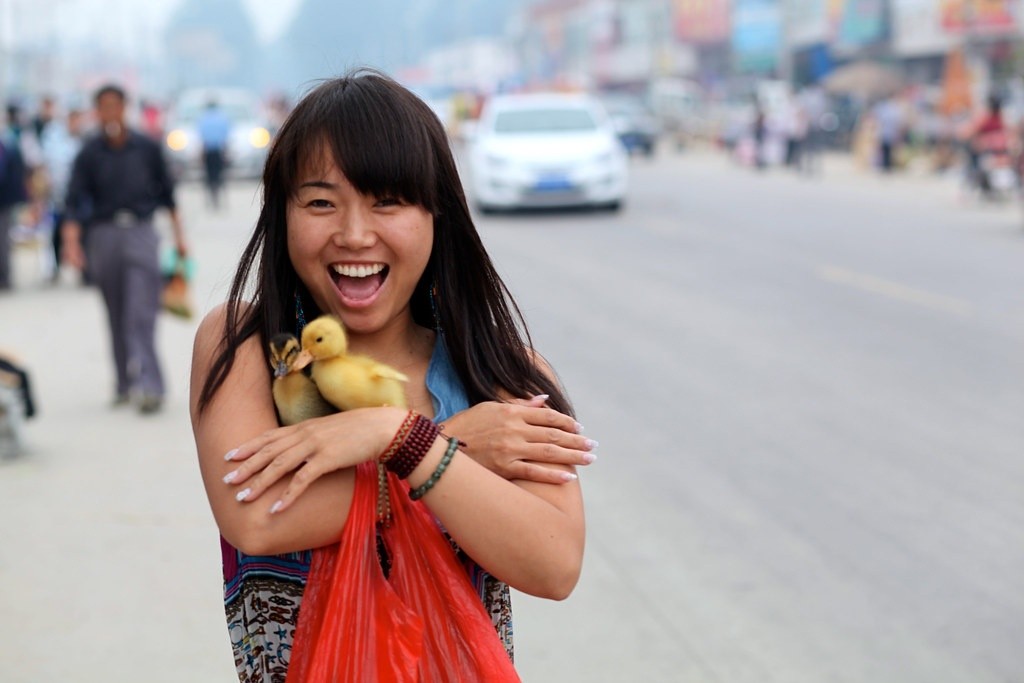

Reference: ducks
[269,334,341,429]
[289,313,408,410]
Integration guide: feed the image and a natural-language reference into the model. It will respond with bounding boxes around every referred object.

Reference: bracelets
[375,461,392,529]
[378,409,468,502]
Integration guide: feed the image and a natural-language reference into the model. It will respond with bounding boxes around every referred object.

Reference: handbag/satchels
[282,465,520,683]
[159,253,196,320]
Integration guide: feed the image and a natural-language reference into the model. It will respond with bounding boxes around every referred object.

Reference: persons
[189,66,598,683]
[0,87,292,290]
[61,87,187,418]
[724,74,1024,199]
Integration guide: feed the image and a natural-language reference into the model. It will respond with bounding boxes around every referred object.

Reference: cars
[152,92,278,180]
[458,93,627,216]
[605,93,659,155]
